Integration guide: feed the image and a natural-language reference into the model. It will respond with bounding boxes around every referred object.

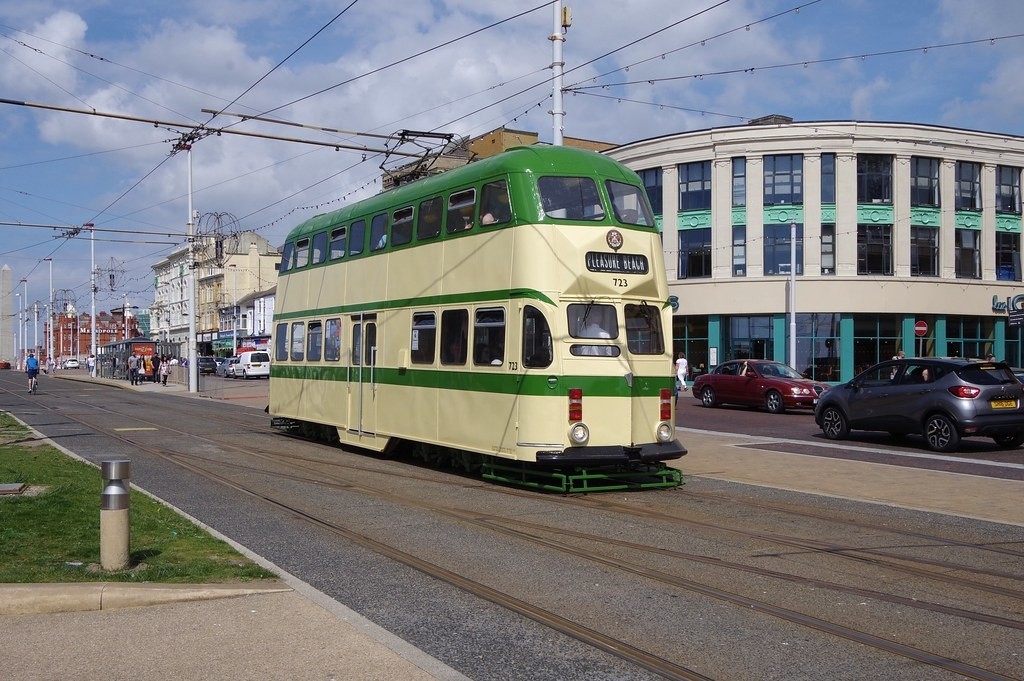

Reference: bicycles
[25,371,38,393]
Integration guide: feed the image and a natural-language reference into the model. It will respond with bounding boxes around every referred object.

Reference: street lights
[44,304,50,359]
[228,264,237,357]
[20,279,27,365]
[43,258,54,360]
[15,293,22,370]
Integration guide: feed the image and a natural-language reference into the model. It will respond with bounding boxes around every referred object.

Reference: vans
[232,351,271,380]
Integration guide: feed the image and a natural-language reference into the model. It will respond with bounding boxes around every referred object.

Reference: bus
[261,128,721,495]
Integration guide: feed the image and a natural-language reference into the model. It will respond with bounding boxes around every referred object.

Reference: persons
[476,344,489,363]
[578,312,612,354]
[922,369,928,382]
[25,354,39,393]
[377,218,406,248]
[54,356,58,367]
[3,360,6,368]
[892,351,909,375]
[161,353,170,363]
[150,352,160,383]
[88,355,95,379]
[465,201,503,228]
[441,337,466,363]
[740,361,749,375]
[128,351,139,386]
[138,354,146,385]
[676,352,689,391]
[197,351,201,356]
[699,363,705,375]
[158,359,170,386]
[987,354,995,361]
[47,354,51,365]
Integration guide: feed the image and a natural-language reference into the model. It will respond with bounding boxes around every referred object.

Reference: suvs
[814,356,1024,453]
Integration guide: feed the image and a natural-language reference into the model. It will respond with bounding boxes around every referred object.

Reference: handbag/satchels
[139,369,146,374]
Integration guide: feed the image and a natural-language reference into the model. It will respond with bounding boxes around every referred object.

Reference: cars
[195,355,239,378]
[692,358,831,414]
[46,357,80,370]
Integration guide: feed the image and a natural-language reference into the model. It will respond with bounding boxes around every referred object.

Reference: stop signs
[915,321,928,337]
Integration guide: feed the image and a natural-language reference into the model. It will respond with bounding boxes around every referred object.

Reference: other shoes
[28,389,32,393]
[36,384,38,387]
[684,387,688,391]
[677,388,681,391]
